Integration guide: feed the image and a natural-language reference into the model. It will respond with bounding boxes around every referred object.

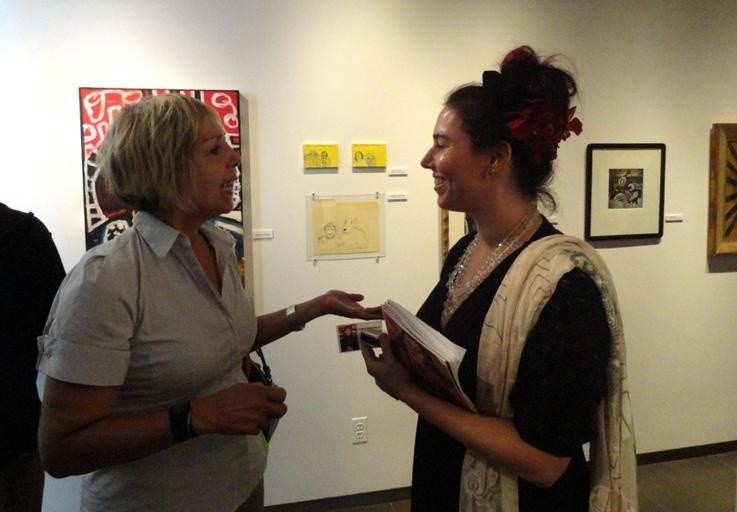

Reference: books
[379,298,478,414]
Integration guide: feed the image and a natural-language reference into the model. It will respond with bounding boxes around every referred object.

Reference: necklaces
[438,211,539,327]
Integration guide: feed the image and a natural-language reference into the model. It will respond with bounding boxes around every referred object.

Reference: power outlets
[350,415,368,445]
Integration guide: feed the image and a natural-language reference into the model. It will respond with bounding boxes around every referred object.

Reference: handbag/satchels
[244,358,282,442]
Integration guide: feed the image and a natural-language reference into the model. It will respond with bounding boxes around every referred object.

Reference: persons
[353,44,643,511]
[31,94,388,510]
[2,203,66,512]
[338,324,360,353]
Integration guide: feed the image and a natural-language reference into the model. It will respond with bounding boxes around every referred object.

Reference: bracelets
[167,401,200,445]
[284,302,307,334]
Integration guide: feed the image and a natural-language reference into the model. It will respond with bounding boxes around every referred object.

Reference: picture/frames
[438,204,474,269]
[705,123,737,257]
[582,140,665,243]
[77,86,245,303]
